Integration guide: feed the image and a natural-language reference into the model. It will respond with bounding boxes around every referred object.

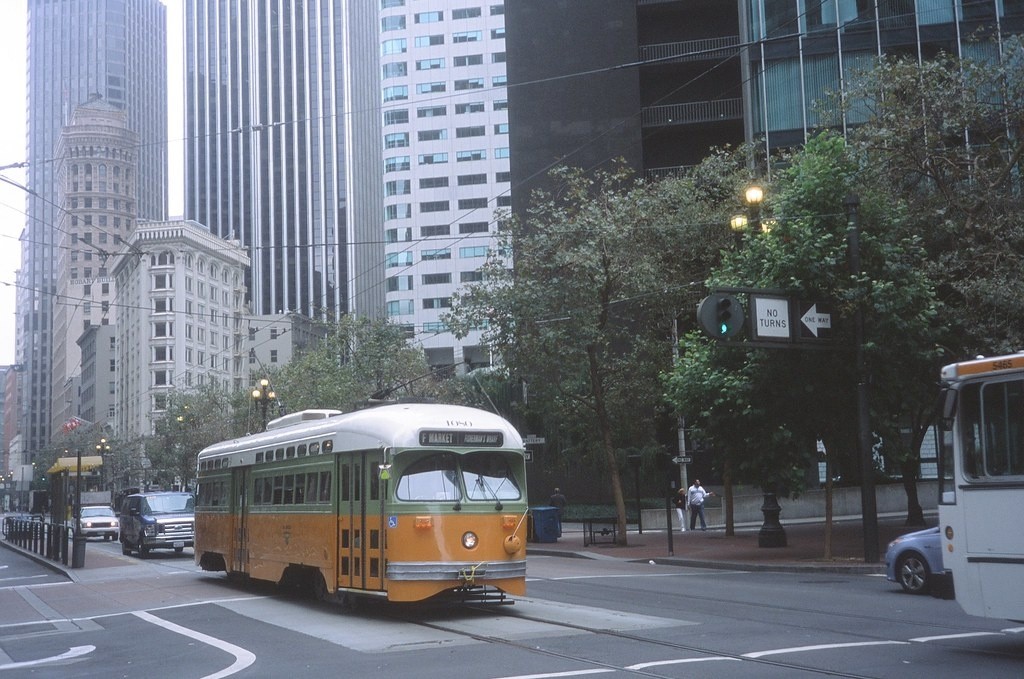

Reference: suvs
[122,492,198,558]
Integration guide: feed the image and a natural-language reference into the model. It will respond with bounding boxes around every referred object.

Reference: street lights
[252,378,275,432]
[724,186,799,547]
[0,449,73,524]
[95,439,111,490]
[176,405,196,491]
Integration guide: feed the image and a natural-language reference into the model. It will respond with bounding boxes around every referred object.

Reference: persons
[687,480,716,532]
[672,488,688,533]
[550,487,566,534]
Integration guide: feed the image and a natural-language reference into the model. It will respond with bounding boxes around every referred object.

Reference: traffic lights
[698,290,746,342]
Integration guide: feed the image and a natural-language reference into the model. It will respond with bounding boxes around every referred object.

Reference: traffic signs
[746,285,844,348]
[670,456,694,464]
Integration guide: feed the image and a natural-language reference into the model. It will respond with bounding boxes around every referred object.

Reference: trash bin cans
[529,506,562,543]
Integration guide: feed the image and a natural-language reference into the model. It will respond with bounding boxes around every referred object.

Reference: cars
[71,506,120,545]
[884,524,957,601]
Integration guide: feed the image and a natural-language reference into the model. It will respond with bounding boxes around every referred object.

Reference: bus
[192,402,529,605]
[933,350,1024,621]
